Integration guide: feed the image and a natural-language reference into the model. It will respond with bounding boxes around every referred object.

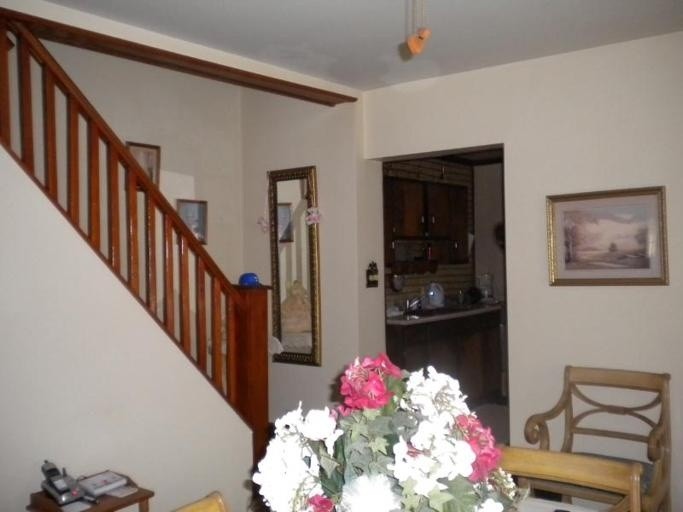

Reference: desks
[26,470,153,512]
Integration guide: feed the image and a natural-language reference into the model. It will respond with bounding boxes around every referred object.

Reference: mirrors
[267,165,322,366]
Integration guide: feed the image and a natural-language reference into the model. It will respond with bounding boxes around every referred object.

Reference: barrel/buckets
[429,280,445,308]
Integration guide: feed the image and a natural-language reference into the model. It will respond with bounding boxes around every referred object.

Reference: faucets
[405,298,422,311]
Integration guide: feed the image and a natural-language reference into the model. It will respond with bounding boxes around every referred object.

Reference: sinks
[391,315,419,321]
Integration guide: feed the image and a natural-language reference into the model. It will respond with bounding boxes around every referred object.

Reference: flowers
[251,354,522,512]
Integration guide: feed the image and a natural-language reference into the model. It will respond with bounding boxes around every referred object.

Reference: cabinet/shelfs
[383,176,471,269]
[401,309,507,408]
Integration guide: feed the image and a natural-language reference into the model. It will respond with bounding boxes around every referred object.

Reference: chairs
[493,365,671,512]
[172,491,227,512]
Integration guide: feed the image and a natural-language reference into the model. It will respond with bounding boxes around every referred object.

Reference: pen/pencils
[83,496,100,505]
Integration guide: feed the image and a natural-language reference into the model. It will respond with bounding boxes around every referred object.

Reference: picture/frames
[545,185,671,287]
[175,198,207,244]
[125,141,160,193]
[276,202,294,242]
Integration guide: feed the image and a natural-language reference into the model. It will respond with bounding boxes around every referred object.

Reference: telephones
[41,460,85,506]
